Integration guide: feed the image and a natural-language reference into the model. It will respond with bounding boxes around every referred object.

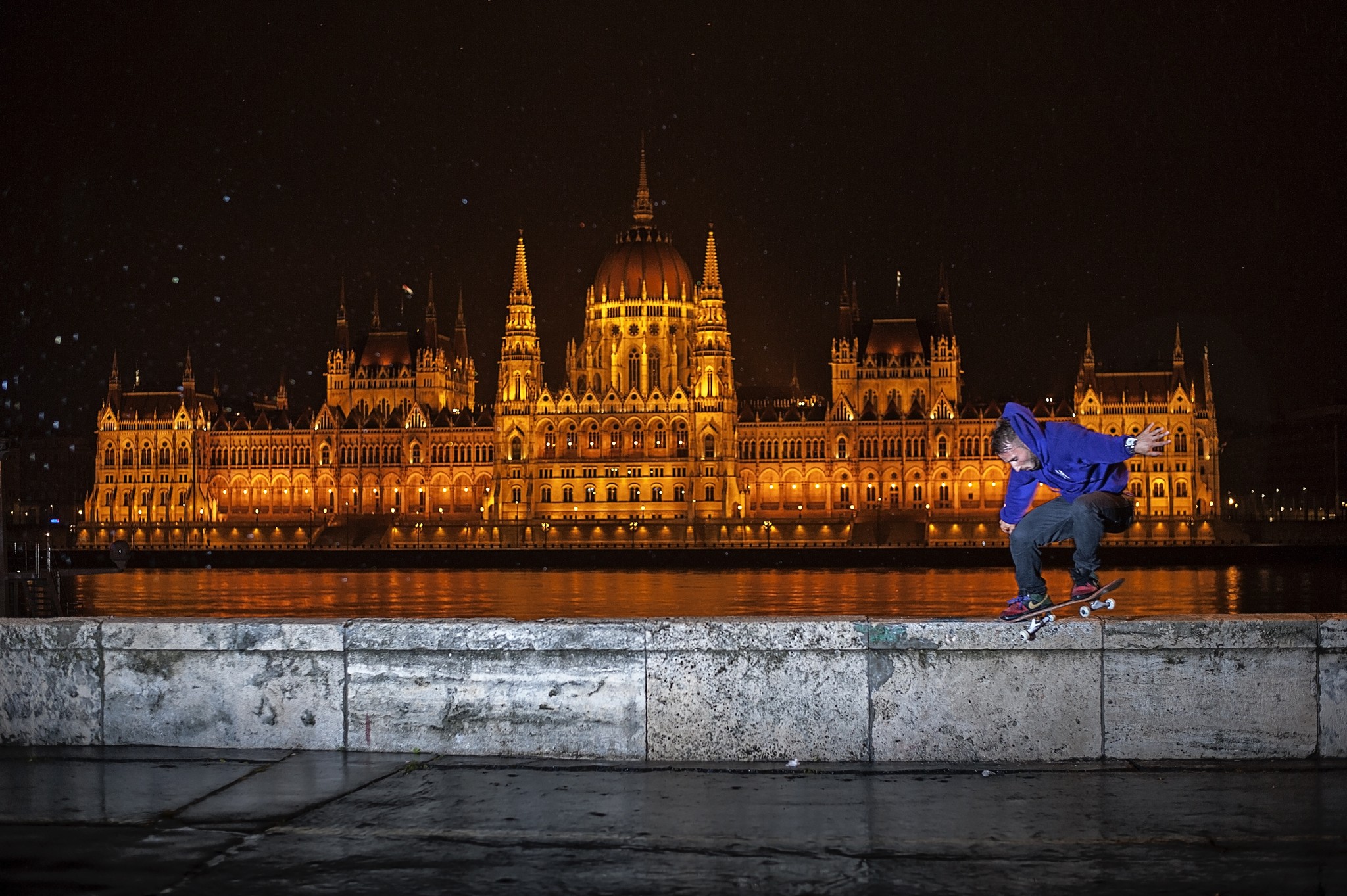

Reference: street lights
[415,523,424,549]
[629,521,639,547]
[132,525,137,549]
[692,499,696,518]
[515,500,519,520]
[541,522,550,549]
[763,520,773,548]
[1185,516,1195,546]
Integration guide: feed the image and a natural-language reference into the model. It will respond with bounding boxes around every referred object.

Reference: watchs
[1125,436,1138,455]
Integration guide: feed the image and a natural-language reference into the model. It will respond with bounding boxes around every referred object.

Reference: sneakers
[1067,566,1100,600]
[998,588,1053,620]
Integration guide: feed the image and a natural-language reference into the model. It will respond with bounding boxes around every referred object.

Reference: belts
[1123,487,1129,492]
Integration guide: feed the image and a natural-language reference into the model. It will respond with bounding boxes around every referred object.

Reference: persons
[991,403,1172,621]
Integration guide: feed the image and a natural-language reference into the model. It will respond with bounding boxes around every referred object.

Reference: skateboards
[998,577,1126,641]
